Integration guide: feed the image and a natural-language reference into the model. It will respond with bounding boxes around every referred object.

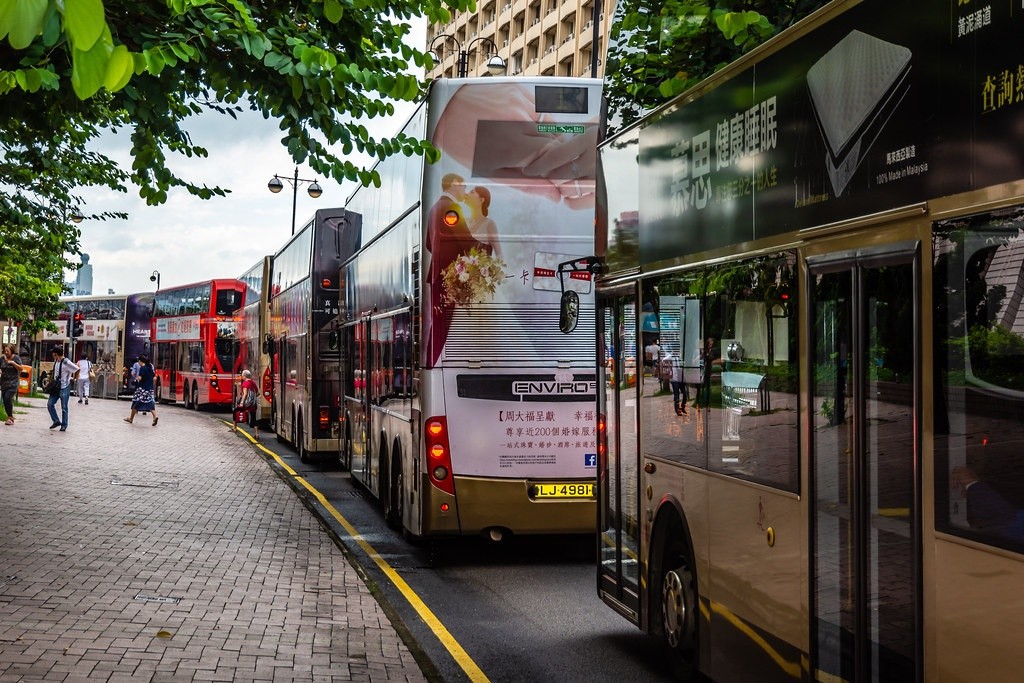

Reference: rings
[537,114,545,124]
[570,161,579,179]
[574,180,582,197]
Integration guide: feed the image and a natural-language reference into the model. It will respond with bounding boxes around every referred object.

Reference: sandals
[228,427,236,432]
[254,434,259,438]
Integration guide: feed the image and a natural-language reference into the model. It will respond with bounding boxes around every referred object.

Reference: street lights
[268,166,323,237]
[150,269,160,290]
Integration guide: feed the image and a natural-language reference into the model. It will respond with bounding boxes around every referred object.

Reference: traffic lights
[73,313,83,337]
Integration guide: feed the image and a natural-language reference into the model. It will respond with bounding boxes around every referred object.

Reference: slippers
[152,417,159,426]
[124,417,133,423]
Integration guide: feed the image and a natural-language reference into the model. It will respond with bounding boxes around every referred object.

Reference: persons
[446,187,539,356]
[76,352,92,405]
[131,352,154,415]
[228,370,259,439]
[46,345,80,432]
[646,338,718,416]
[425,175,493,370]
[428,82,570,203]
[124,354,158,426]
[0,343,23,424]
[521,115,600,210]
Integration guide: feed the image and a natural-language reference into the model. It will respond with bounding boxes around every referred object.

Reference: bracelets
[241,403,244,404]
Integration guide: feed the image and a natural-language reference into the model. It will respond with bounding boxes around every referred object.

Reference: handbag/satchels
[131,379,139,386]
[237,388,255,407]
[236,411,248,423]
[44,377,60,395]
[88,361,95,380]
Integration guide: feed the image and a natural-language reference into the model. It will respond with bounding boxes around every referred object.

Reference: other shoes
[143,412,146,414]
[50,421,61,428]
[681,407,687,413]
[675,408,683,415]
[60,425,67,431]
[6,417,14,425]
[78,399,82,402]
[85,400,88,404]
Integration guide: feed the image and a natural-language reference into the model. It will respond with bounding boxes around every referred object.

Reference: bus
[558,0,1024,683]
[262,206,346,466]
[0,255,272,428]
[328,76,603,545]
